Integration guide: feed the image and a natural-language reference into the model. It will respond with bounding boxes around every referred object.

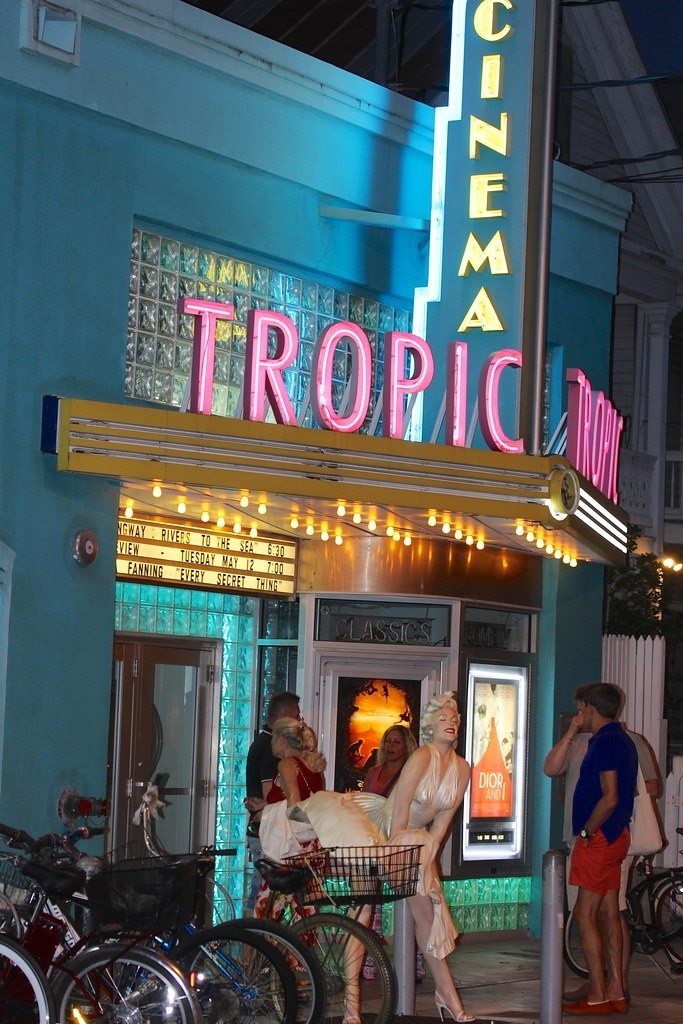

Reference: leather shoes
[562,997,613,1017]
[613,997,630,1013]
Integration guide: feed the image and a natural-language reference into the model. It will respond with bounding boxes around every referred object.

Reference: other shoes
[624,991,631,1002]
[562,982,592,1002]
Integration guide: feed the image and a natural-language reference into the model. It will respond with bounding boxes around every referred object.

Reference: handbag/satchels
[627,793,663,856]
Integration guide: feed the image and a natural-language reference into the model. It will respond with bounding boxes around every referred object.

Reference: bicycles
[1,770,425,1024]
[562,827,683,983]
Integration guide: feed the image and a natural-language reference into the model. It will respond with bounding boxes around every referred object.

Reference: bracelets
[563,735,572,744]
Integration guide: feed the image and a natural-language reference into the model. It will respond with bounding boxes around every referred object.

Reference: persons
[343,725,426,984]
[296,691,476,1024]
[242,691,322,1000]
[543,684,659,1015]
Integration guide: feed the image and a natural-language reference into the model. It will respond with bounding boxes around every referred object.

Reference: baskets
[0,852,48,918]
[112,852,203,930]
[281,844,422,908]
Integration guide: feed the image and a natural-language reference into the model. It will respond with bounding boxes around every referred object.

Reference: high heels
[342,1016,362,1024]
[434,990,476,1022]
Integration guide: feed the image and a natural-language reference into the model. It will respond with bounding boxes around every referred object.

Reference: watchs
[580,829,592,839]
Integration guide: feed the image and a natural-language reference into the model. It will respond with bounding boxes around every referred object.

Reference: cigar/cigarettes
[250,820,254,823]
